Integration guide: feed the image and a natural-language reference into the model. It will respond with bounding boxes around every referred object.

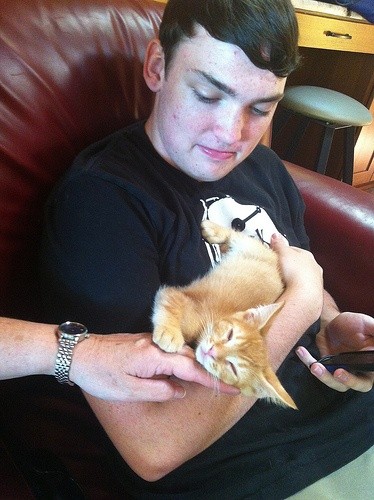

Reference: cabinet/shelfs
[260,0,374,188]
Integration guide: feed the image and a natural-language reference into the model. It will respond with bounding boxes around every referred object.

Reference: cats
[151,220,300,411]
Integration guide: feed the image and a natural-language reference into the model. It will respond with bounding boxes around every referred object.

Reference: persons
[38,0,374,500]
[0,315,241,403]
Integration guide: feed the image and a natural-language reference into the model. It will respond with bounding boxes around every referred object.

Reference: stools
[272,85,373,186]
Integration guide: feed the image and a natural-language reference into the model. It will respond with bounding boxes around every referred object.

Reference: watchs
[54,321,90,385]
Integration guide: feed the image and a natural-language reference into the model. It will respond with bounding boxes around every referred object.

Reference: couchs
[0,0,374,500]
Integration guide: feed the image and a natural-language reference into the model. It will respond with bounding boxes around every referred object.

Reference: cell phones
[310,350,374,372]
